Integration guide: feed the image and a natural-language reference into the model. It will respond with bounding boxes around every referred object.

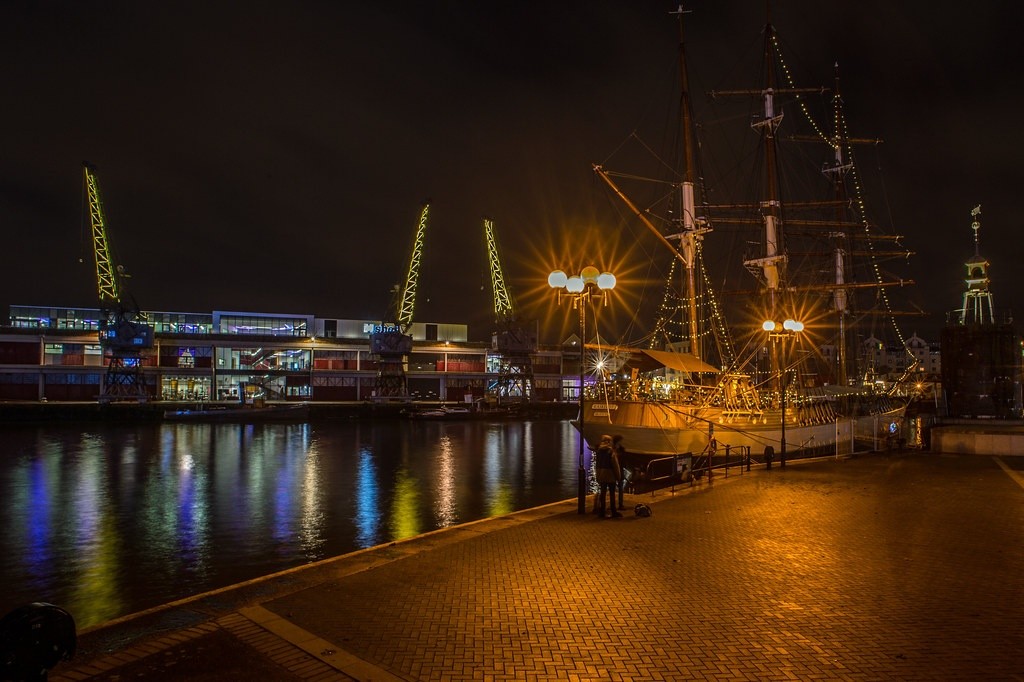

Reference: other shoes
[599,512,605,517]
[612,511,623,517]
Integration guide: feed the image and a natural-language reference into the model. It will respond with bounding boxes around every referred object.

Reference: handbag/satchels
[635,503,652,516]
[594,492,602,513]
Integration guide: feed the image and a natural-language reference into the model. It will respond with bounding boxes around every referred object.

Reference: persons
[596,435,622,517]
[606,435,625,510]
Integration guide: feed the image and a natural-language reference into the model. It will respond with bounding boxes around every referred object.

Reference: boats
[163,393,545,424]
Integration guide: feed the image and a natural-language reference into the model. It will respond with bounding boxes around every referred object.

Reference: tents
[642,350,722,373]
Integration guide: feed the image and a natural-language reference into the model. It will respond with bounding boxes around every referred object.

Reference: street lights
[763,318,805,468]
[547,266,617,516]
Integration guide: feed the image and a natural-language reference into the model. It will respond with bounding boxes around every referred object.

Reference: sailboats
[569,13,937,492]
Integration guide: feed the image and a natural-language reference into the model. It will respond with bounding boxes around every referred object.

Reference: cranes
[362,201,431,402]
[84,166,155,406]
[482,218,539,405]
